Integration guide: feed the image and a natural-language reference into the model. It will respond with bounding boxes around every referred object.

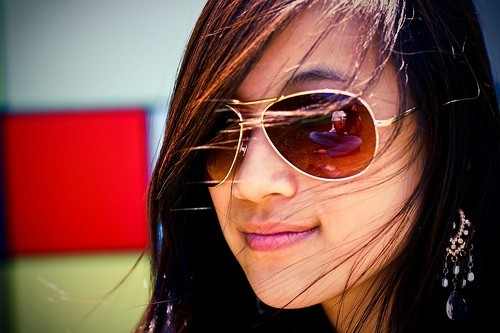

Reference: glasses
[195,88,481,190]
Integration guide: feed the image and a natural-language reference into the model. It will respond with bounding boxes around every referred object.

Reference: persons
[310,93,366,157]
[134,1,500,333]
